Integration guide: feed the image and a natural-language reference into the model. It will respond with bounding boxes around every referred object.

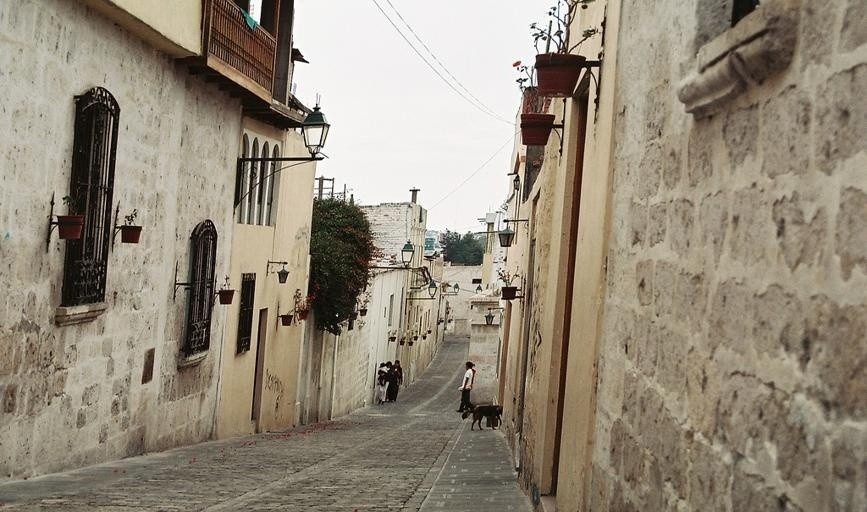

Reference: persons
[456,362,473,412]
[377,359,402,406]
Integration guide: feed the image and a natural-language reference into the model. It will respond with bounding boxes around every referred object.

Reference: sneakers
[379,400,384,404]
[457,407,467,412]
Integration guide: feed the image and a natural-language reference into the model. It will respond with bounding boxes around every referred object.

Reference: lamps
[485,307,505,326]
[476,284,482,294]
[498,219,529,247]
[405,280,459,315]
[363,238,415,292]
[267,260,290,284]
[232,102,332,215]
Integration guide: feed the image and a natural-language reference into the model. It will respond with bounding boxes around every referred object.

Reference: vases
[360,309,367,315]
[520,114,555,146]
[281,315,293,326]
[299,309,309,319]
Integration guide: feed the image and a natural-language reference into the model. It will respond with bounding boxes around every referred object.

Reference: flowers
[513,59,543,113]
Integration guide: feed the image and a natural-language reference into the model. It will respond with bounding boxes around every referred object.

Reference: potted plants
[121,208,143,243]
[495,266,520,299]
[391,326,432,346]
[57,186,84,240]
[528,1,603,98]
[219,275,234,304]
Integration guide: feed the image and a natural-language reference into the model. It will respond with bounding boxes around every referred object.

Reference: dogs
[462,405,503,431]
